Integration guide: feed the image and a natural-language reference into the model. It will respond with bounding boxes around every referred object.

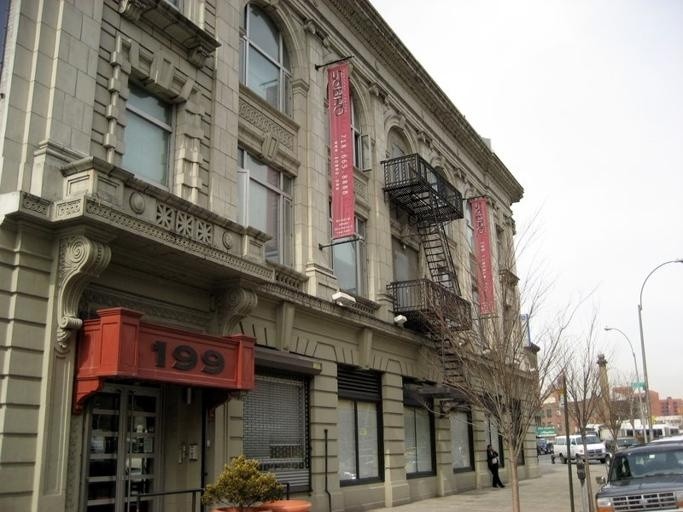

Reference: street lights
[603,260,683,444]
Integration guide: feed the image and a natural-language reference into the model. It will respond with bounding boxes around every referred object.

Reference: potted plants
[201,455,284,512]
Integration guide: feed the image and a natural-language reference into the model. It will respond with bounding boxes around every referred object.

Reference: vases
[262,500,312,512]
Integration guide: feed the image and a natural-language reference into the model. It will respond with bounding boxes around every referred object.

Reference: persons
[486,443,506,489]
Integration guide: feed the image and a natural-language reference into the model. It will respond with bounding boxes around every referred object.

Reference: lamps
[332,293,356,308]
[178,441,197,465]
[395,315,407,329]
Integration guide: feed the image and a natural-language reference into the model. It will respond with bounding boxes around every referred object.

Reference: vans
[537,415,683,464]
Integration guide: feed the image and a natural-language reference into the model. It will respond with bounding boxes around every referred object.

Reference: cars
[595,441,683,512]
